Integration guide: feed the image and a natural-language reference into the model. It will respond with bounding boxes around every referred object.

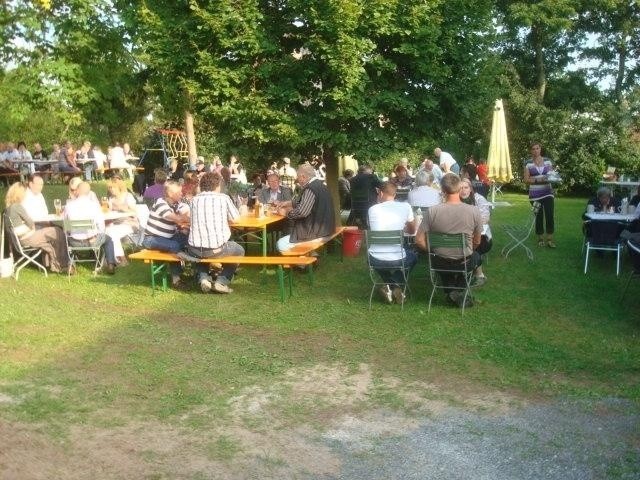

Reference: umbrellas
[486,98,514,203]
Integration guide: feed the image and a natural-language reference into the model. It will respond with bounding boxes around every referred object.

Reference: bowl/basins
[547,172,560,178]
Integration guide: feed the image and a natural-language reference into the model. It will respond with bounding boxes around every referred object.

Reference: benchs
[127,251,317,303]
[0,167,145,186]
[281,226,345,287]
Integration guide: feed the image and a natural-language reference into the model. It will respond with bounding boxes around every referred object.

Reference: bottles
[620,175,623,183]
[255,200,264,218]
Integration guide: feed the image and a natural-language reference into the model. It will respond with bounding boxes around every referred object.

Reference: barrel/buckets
[342,228,365,257]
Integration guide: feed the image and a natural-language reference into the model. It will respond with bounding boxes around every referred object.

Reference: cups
[618,205,628,214]
[264,203,273,217]
[238,205,251,216]
[53,199,62,215]
[102,197,109,212]
[627,179,630,183]
[586,205,594,213]
[629,206,635,213]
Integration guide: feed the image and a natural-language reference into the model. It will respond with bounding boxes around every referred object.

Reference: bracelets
[535,178,537,184]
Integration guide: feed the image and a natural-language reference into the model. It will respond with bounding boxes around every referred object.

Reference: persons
[339,147,490,307]
[62,174,139,275]
[142,155,247,293]
[523,141,556,248]
[0,142,136,187]
[583,185,640,257]
[5,175,73,272]
[249,158,334,272]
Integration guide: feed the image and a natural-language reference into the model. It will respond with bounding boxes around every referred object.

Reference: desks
[11,156,141,167]
[599,181,640,196]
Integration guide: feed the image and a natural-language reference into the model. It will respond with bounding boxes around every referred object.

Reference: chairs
[499,201,542,259]
[363,229,413,311]
[63,217,106,283]
[620,239,640,304]
[395,189,410,201]
[582,219,624,278]
[425,231,476,318]
[3,213,48,281]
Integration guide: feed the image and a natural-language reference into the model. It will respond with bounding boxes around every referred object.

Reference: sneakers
[173,280,191,291]
[393,288,407,304]
[379,285,393,304]
[547,239,557,248]
[214,281,233,293]
[107,263,115,274]
[474,276,487,287]
[200,279,212,293]
[449,290,475,307]
[538,239,546,247]
